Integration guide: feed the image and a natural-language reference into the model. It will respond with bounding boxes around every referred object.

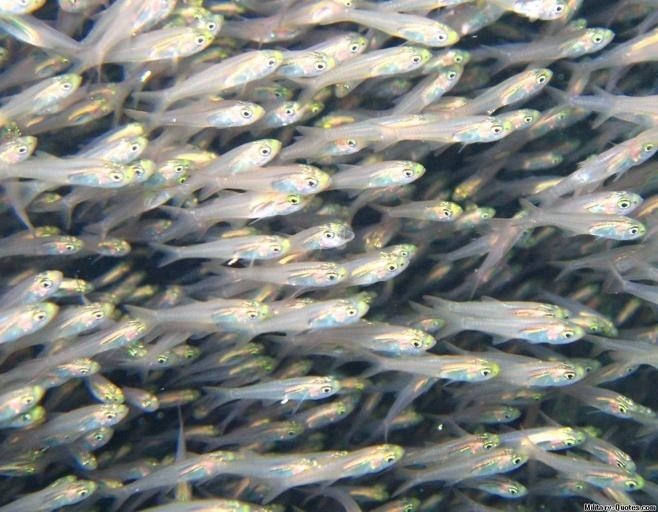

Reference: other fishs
[0,0,658,512]
